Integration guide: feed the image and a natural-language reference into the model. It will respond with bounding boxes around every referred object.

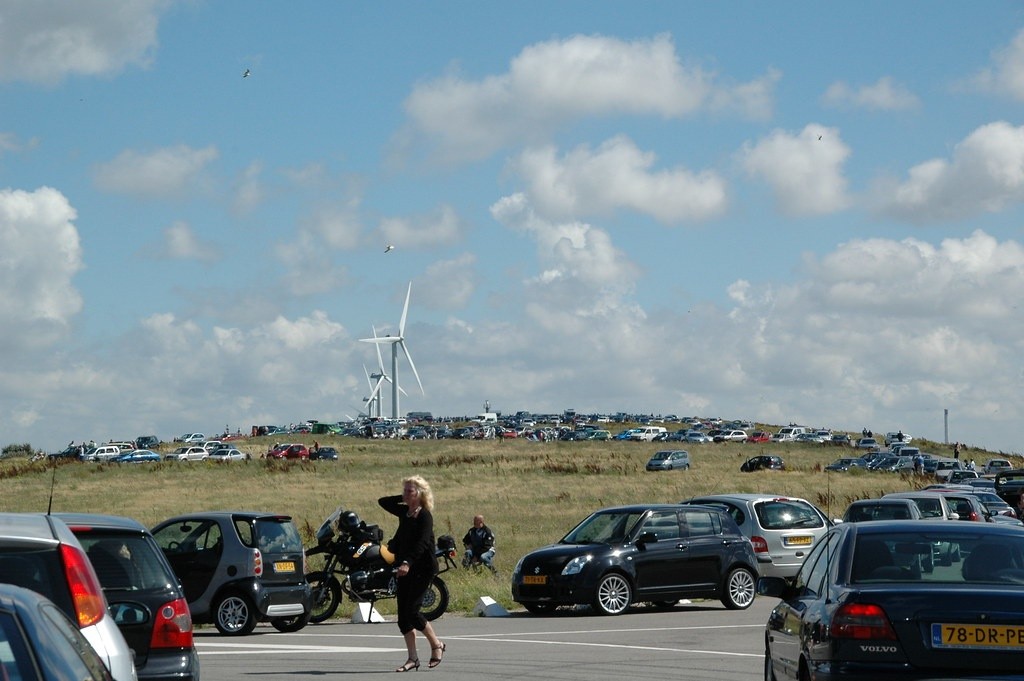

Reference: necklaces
[406,506,419,518]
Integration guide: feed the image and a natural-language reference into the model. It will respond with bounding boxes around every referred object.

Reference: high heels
[397,657,421,673]
[428,643,447,668]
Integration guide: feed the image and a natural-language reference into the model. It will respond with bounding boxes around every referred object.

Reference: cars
[882,491,962,567]
[266,443,309,462]
[713,430,749,445]
[257,408,756,444]
[708,429,726,438]
[176,432,206,444]
[740,455,785,473]
[794,433,824,445]
[769,432,795,442]
[207,449,248,463]
[308,447,339,461]
[135,435,160,450]
[511,503,761,617]
[832,434,849,447]
[148,511,313,639]
[198,440,234,456]
[109,449,160,464]
[815,430,833,442]
[764,520,1024,681]
[746,432,770,445]
[0,582,118,681]
[214,432,241,442]
[48,439,137,461]
[839,499,934,580]
[661,493,836,596]
[824,458,869,473]
[859,438,880,452]
[862,431,1024,557]
[40,510,200,681]
[0,510,139,681]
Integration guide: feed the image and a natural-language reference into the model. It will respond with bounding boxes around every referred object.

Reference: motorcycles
[302,507,460,625]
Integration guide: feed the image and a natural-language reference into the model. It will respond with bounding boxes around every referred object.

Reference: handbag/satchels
[388,504,408,554]
[437,535,456,551]
[337,521,384,546]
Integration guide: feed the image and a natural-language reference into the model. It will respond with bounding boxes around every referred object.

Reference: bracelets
[403,560,408,563]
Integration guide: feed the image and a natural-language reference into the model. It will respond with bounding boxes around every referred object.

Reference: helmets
[339,510,360,534]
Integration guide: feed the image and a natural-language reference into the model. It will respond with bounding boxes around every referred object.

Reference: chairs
[1006,477,1013,481]
[735,510,745,526]
[934,463,937,467]
[1001,462,1008,466]
[962,543,1015,582]
[987,496,992,501]
[893,511,907,519]
[918,501,937,512]
[938,464,946,470]
[0,556,42,595]
[953,474,961,479]
[86,545,142,590]
[640,521,653,536]
[857,511,873,521]
[851,540,896,580]
[953,464,959,469]
[946,466,953,469]
[957,504,970,512]
[654,521,676,539]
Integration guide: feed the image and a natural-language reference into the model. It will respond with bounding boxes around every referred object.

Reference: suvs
[163,447,209,462]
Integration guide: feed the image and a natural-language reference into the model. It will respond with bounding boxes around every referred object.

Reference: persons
[309,441,319,459]
[492,426,505,442]
[867,430,872,437]
[436,416,442,423]
[898,431,903,441]
[274,442,279,449]
[789,422,797,426]
[954,449,959,458]
[378,475,445,672]
[863,427,867,437]
[829,429,833,436]
[462,515,497,575]
[964,458,975,471]
[525,428,566,442]
[912,455,924,475]
[846,432,850,440]
[67,440,96,459]
[109,439,113,443]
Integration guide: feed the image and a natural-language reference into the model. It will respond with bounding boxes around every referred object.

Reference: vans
[645,449,690,471]
[778,427,806,442]
[82,445,121,463]
